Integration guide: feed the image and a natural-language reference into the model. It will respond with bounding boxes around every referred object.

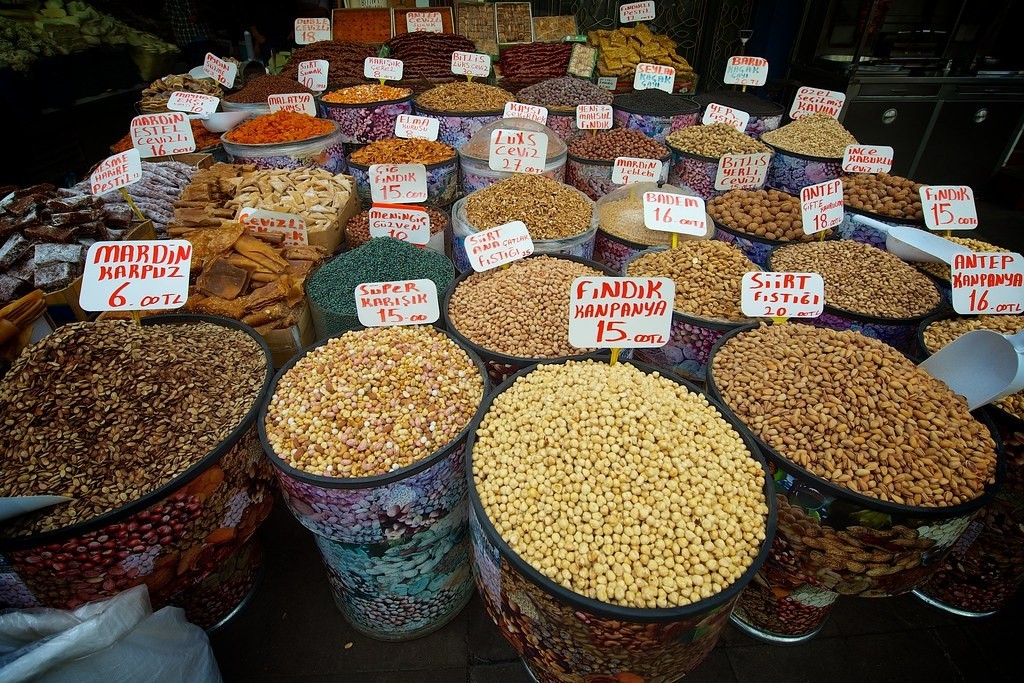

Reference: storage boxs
[0,153,360,369]
[330,2,579,46]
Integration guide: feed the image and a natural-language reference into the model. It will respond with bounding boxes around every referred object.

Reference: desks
[0,44,145,191]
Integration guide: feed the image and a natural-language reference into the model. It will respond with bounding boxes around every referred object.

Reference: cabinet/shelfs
[776,80,1024,195]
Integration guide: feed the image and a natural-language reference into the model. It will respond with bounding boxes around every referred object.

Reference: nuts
[0,77,1023,611]
